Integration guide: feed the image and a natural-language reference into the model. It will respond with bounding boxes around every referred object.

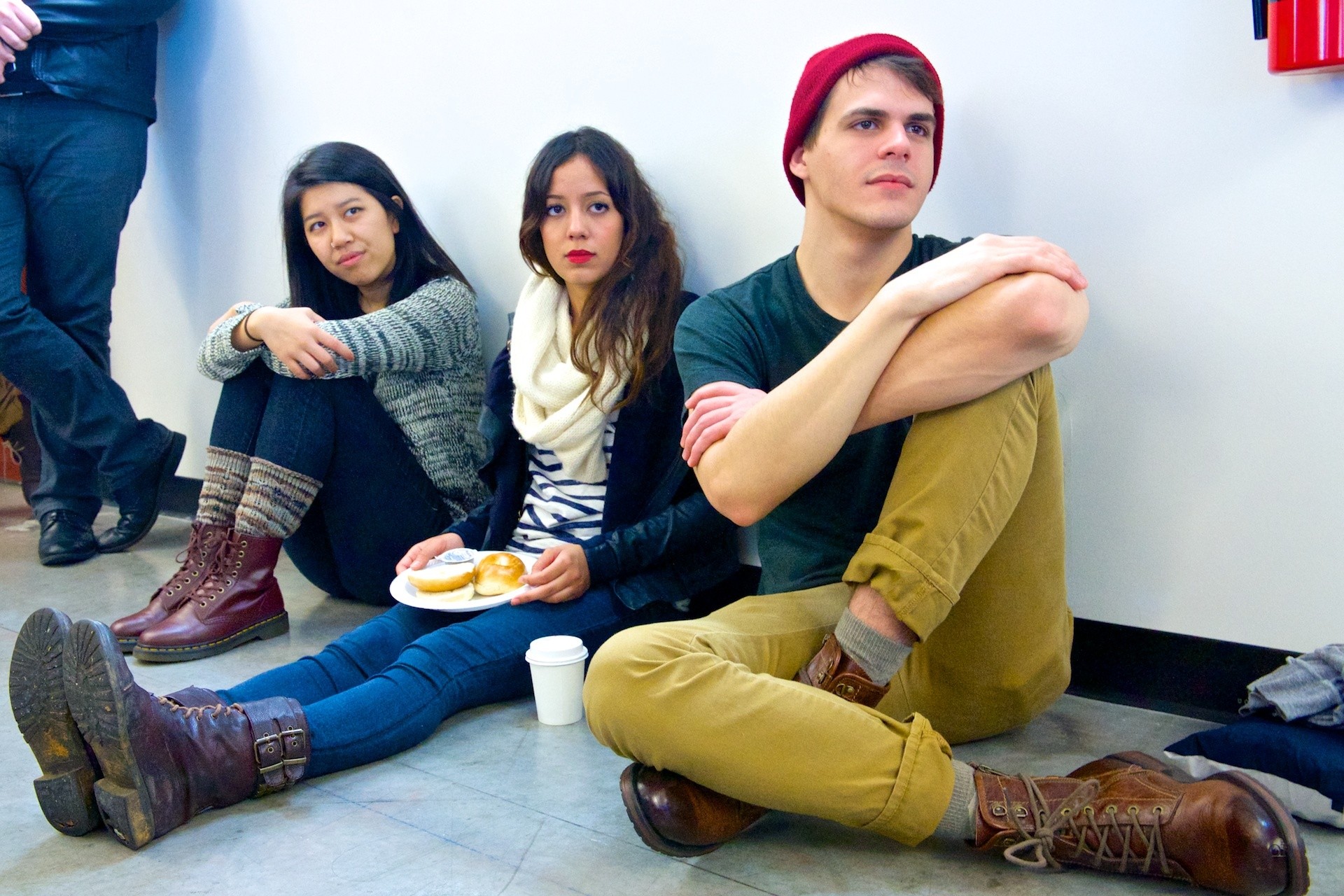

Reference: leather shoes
[37,508,96,567]
[95,430,190,552]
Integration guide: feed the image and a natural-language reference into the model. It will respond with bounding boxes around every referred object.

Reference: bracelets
[243,306,264,343]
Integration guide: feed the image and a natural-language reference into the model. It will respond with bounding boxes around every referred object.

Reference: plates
[390,550,541,614]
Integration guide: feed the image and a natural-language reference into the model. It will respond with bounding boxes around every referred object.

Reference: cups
[525,635,588,726]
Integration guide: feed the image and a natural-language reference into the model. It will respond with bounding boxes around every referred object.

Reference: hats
[783,33,943,206]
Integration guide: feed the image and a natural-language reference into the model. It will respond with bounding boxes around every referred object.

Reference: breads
[405,552,527,605]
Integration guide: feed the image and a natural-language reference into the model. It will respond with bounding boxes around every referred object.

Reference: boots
[60,620,310,851]
[10,608,229,839]
[133,530,289,662]
[620,629,891,860]
[966,749,1311,894]
[108,521,236,653]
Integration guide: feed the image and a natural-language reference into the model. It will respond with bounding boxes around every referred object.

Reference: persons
[579,30,1320,895]
[103,141,495,667]
[0,0,191,570]
[5,122,752,854]
